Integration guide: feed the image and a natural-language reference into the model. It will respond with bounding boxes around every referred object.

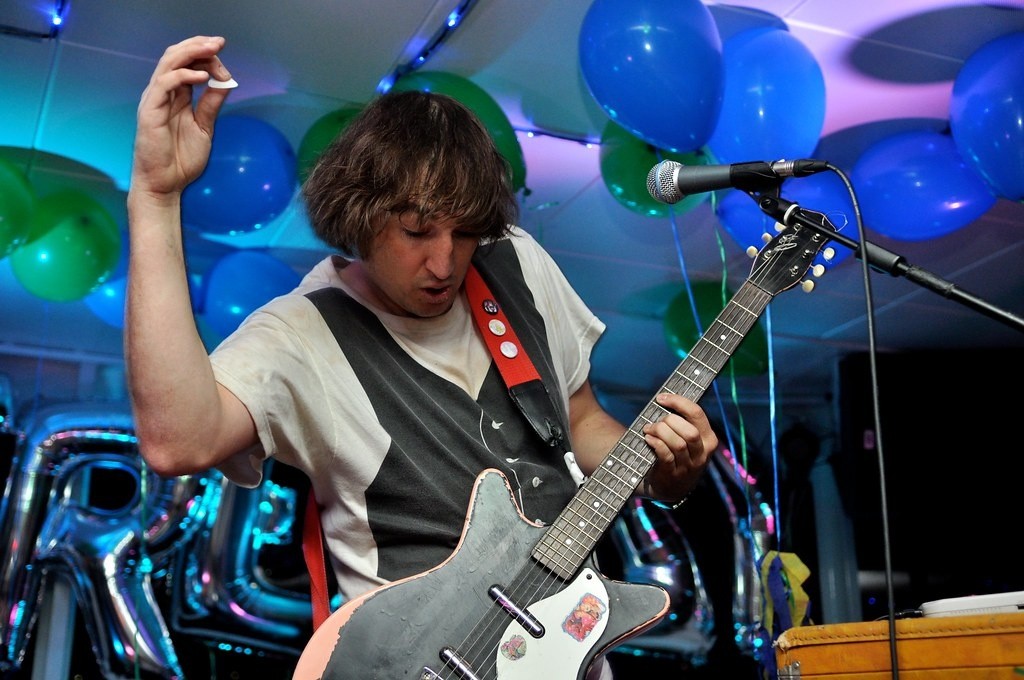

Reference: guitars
[291,207,837,679]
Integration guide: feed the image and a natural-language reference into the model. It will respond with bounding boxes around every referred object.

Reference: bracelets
[644,478,695,513]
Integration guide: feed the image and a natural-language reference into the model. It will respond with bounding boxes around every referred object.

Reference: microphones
[647,160,829,205]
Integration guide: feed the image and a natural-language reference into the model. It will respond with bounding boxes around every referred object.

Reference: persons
[124,34,717,680]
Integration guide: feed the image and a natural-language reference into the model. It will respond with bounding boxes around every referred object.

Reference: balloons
[0,0,1024,390]
